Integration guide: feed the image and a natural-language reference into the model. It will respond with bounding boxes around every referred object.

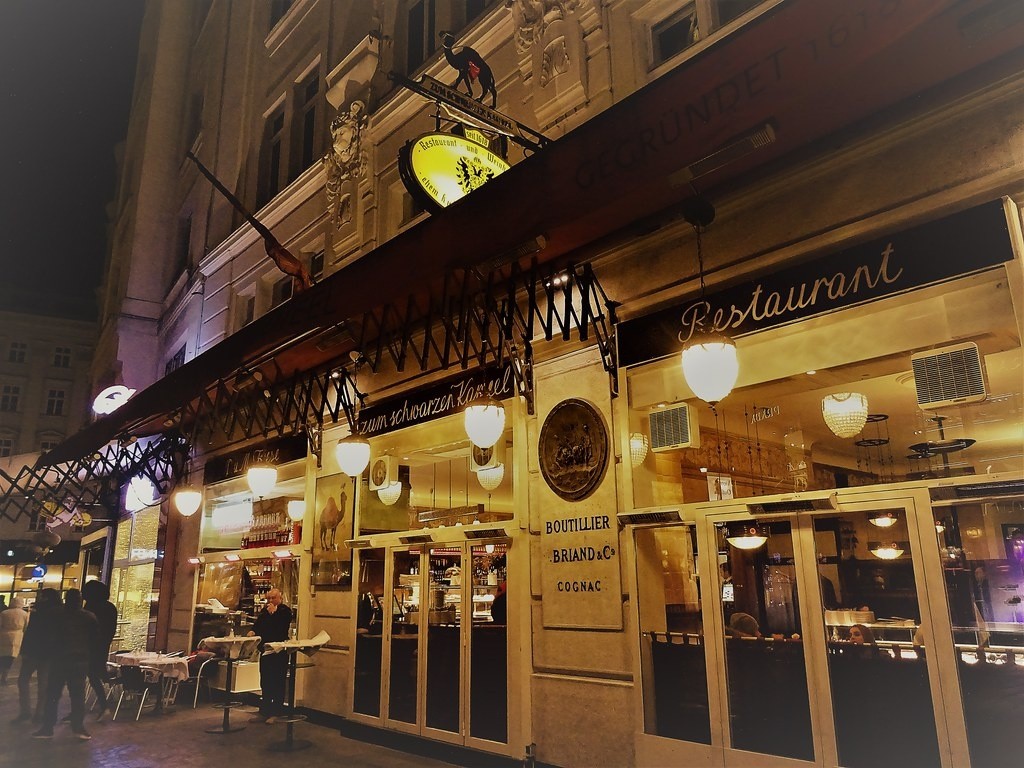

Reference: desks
[114,651,188,709]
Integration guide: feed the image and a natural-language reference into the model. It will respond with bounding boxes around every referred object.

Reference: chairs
[84,657,164,722]
[160,648,216,710]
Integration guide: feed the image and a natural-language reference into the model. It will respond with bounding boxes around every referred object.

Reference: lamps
[868,511,944,560]
[175,446,202,518]
[465,263,506,491]
[822,392,868,438]
[246,339,402,521]
[725,521,772,548]
[680,195,738,409]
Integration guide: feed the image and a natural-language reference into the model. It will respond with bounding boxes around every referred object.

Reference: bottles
[248,559,272,616]
[158,651,162,660]
[240,513,300,550]
[229,628,234,638]
[292,629,297,641]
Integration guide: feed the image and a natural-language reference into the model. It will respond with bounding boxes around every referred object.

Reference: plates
[851,610,874,625]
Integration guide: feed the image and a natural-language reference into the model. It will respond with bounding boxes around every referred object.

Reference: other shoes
[265,715,281,724]
[62,712,73,723]
[250,715,270,722]
[10,712,32,724]
[96,705,111,722]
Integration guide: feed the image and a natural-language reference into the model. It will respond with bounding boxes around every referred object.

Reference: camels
[440,31,497,109]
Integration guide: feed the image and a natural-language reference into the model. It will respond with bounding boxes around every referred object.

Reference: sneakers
[72,726,91,739]
[32,727,54,739]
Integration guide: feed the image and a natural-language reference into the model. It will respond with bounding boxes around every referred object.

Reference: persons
[720,562,736,596]
[11,579,98,740]
[0,595,9,612]
[724,614,763,637]
[848,624,875,644]
[491,580,507,625]
[0,597,29,683]
[247,587,292,726]
[83,581,117,722]
[357,623,385,634]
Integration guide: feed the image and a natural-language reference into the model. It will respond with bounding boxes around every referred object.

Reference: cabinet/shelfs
[239,559,274,615]
[394,584,497,621]
